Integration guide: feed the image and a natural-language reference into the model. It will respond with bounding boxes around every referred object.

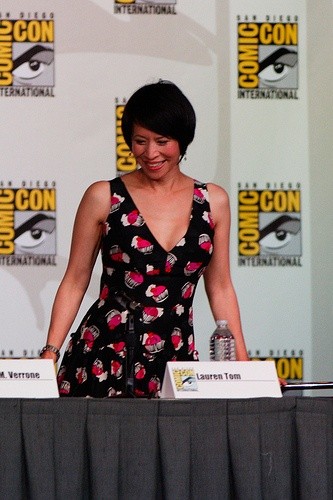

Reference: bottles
[209,319,236,361]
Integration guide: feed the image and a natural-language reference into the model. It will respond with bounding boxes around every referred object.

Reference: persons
[39,80,287,398]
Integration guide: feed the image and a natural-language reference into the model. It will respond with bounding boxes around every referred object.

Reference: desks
[0,396,333,500]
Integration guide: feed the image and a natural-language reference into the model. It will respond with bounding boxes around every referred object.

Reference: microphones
[108,287,144,313]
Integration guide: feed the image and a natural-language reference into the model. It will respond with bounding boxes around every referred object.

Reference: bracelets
[40,344,61,362]
